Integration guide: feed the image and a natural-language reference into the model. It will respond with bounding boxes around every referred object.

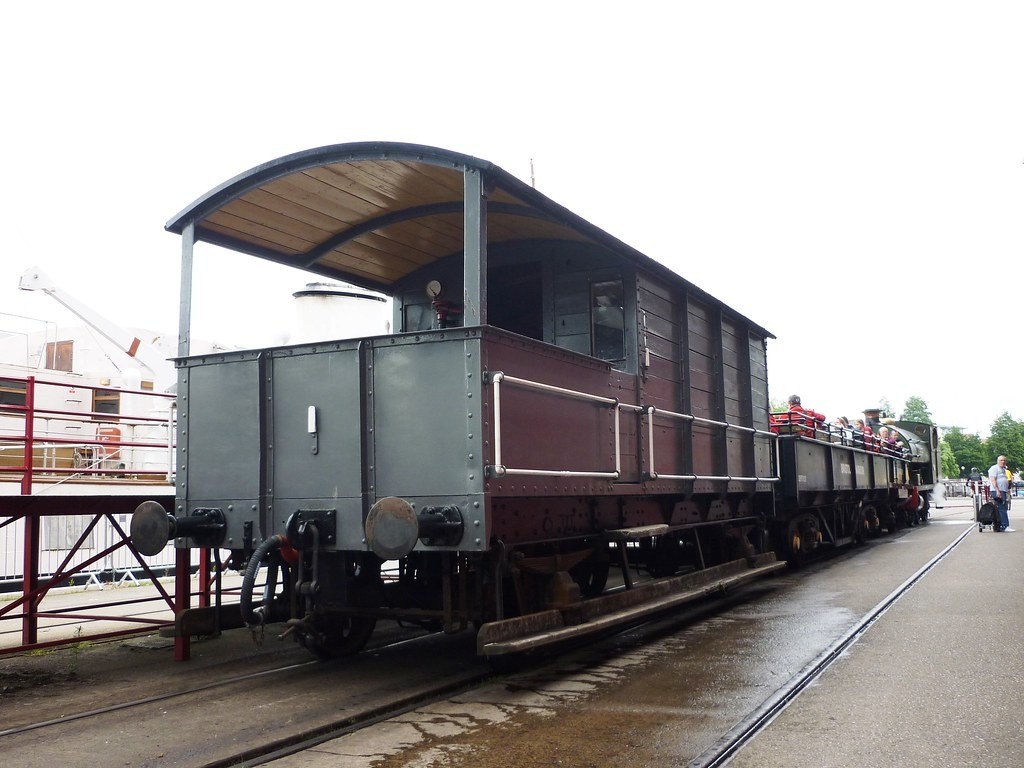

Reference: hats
[789,395,800,404]
[838,417,849,425]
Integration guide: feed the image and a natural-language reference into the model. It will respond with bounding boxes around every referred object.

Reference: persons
[988,455,1017,532]
[770,394,912,459]
[965,467,983,498]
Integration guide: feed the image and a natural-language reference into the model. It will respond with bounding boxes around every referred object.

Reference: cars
[1011,472,1024,497]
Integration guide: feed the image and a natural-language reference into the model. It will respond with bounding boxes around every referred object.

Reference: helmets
[971,467,978,472]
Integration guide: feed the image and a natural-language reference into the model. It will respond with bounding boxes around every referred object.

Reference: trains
[128,140,943,682]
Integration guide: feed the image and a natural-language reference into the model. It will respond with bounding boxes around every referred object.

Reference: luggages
[977,498,998,532]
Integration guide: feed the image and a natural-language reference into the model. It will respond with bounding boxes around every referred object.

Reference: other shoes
[1005,526,1016,532]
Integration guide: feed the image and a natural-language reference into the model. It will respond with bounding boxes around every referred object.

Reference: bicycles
[967,483,983,507]
